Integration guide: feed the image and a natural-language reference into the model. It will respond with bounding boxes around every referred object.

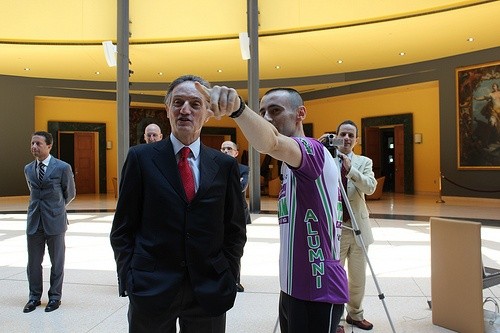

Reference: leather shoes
[346,313,373,330]
[45,300,60,312]
[23,298,41,313]
[236,282,244,292]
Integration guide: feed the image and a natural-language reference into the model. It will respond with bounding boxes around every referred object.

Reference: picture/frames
[455,61,500,171]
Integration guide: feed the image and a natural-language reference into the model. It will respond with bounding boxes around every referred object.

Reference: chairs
[112,177,117,198]
[430,218,500,333]
[269,177,281,197]
[365,176,386,200]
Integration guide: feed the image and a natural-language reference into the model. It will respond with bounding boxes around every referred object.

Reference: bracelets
[230,95,245,118]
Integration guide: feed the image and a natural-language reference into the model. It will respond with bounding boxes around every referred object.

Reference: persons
[110,74,247,333]
[318,120,378,333]
[193,81,348,333]
[144,124,163,144]
[220,141,251,292]
[23,131,76,313]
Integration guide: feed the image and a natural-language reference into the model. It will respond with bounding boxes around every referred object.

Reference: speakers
[102,40,117,67]
[239,32,250,60]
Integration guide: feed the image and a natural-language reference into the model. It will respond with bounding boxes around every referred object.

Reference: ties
[37,163,44,184]
[179,146,195,202]
[341,163,351,222]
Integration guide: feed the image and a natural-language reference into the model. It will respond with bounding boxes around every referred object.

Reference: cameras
[323,134,344,147]
